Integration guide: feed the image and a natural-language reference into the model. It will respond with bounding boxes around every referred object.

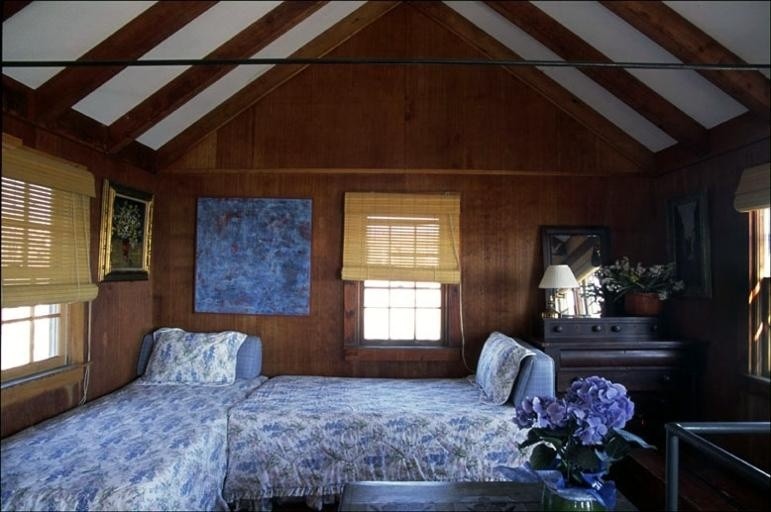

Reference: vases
[558,438,606,489]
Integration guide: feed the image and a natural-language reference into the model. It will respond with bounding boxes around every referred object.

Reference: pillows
[465,331,538,406]
[133,327,248,386]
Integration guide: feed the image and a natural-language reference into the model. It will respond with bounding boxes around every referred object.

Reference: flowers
[515,375,661,459]
[594,254,688,301]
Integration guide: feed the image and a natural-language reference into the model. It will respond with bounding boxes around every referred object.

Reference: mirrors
[542,225,608,318]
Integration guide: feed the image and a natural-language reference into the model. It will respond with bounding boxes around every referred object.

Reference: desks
[537,316,692,425]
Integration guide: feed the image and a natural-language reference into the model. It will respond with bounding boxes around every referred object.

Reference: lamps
[539,264,579,318]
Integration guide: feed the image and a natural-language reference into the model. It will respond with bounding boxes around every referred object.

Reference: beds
[1,328,267,512]
[226,331,556,512]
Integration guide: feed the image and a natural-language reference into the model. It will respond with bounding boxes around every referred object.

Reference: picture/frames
[665,187,714,300]
[96,178,152,282]
